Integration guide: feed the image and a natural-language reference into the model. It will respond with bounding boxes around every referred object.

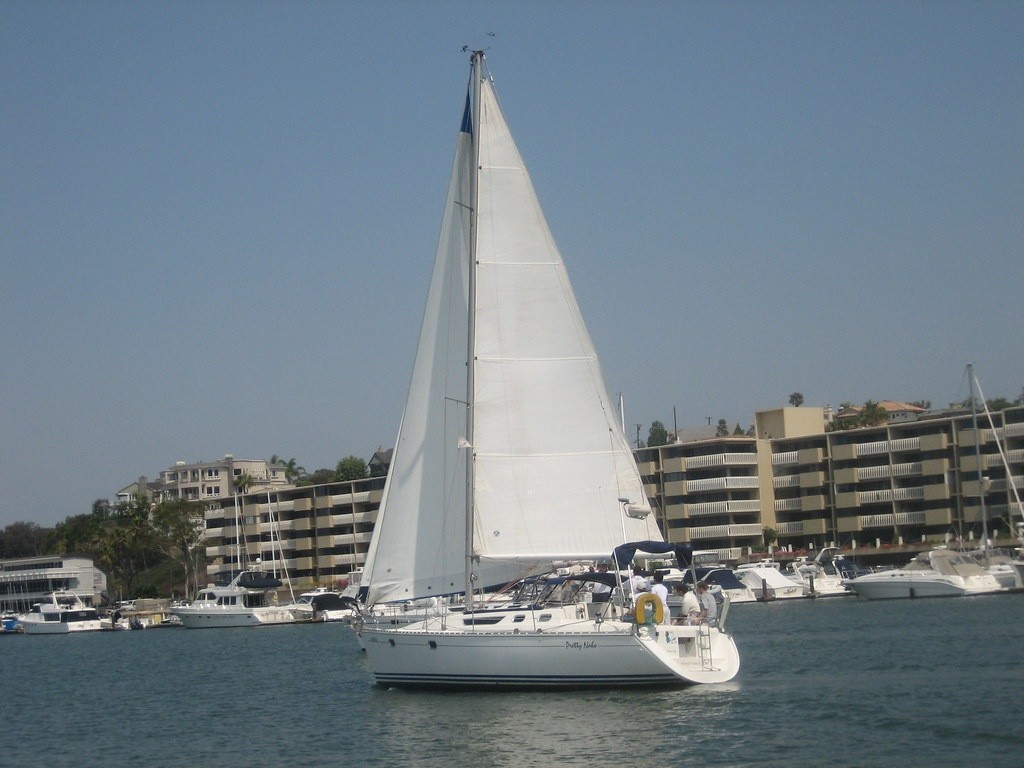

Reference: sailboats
[352,54,741,699]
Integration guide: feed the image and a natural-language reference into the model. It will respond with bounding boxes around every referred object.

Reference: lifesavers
[634,592,664,625]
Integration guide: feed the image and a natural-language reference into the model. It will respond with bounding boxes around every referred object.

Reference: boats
[0,476,358,636]
[622,363,1024,600]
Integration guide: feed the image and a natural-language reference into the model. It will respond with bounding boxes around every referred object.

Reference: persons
[547,560,717,625]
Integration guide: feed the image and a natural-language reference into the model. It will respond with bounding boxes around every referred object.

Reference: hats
[675,584,688,593]
[695,580,708,587]
[597,560,608,567]
[636,582,647,590]
